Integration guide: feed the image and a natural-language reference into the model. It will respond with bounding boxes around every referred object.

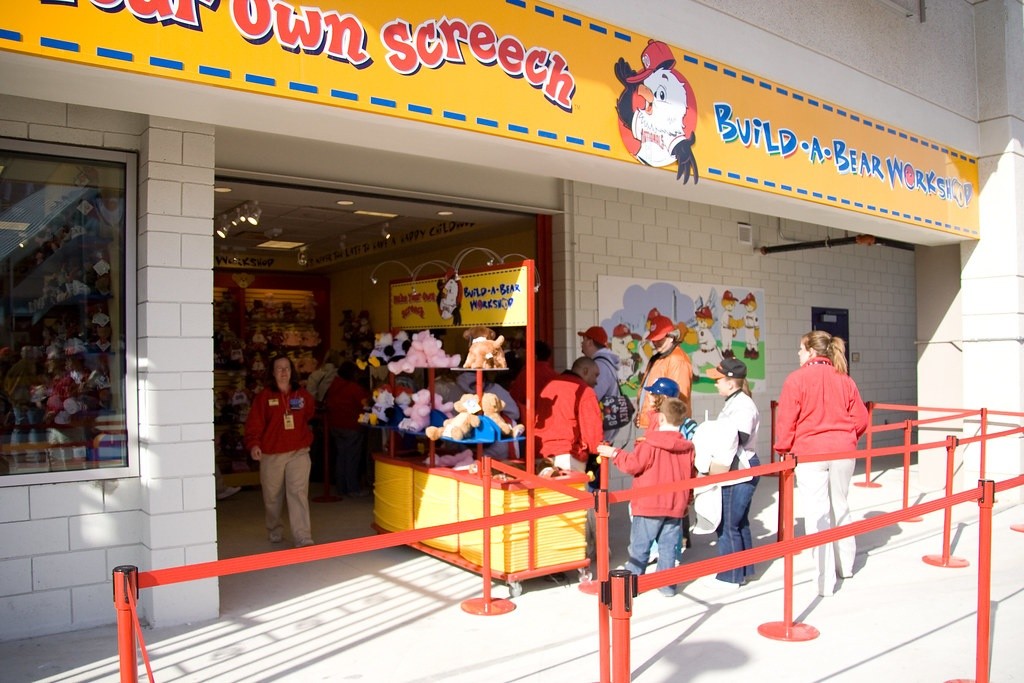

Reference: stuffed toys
[215,291,323,472]
[355,328,526,442]
[30,225,114,352]
[338,308,374,361]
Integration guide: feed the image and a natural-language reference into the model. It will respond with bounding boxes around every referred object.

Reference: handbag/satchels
[588,359,635,431]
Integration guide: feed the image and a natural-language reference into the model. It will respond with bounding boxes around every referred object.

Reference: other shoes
[269,526,283,543]
[217,486,241,500]
[707,579,740,593]
[545,573,566,582]
[295,539,314,548]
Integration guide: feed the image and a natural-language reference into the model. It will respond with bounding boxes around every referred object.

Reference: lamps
[217,226,228,239]
[247,206,262,226]
[231,214,240,227]
[239,210,249,223]
[222,221,231,231]
[380,230,390,240]
[297,252,308,266]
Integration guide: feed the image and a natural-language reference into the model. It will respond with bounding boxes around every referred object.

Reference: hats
[646,316,674,341]
[577,326,608,347]
[706,356,746,379]
[644,377,680,398]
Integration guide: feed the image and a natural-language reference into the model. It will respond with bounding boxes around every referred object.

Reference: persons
[596,378,699,599]
[321,362,376,496]
[305,349,340,405]
[708,357,761,590]
[509,340,558,436]
[493,352,523,389]
[632,315,694,429]
[772,329,870,595]
[0,344,114,471]
[216,466,242,500]
[577,326,623,519]
[244,354,314,548]
[529,355,611,561]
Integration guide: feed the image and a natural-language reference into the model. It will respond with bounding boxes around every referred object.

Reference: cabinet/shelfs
[32,187,115,356]
[243,317,324,379]
